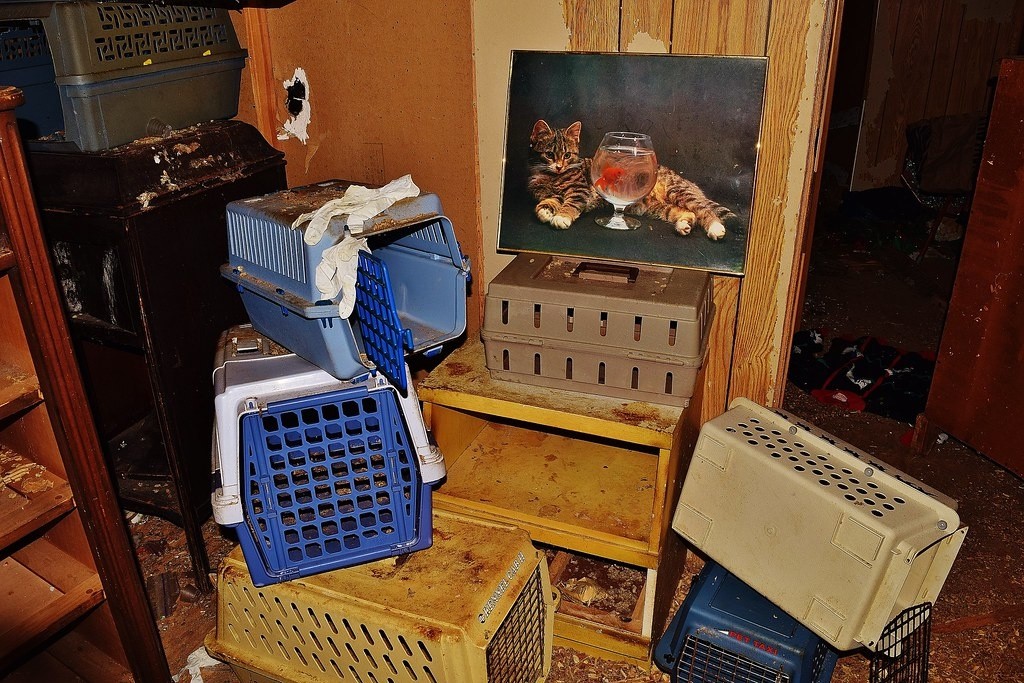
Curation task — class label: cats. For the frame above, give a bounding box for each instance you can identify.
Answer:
[521,119,737,242]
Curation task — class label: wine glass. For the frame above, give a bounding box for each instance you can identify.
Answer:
[590,131,658,230]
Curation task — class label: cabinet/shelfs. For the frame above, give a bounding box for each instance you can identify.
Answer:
[821,1,1024,192]
[1,83,174,683]
[20,117,288,594]
[418,329,710,677]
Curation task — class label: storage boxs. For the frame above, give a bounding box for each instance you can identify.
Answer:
[0,0,251,154]
[203,508,555,683]
[654,397,969,658]
[652,559,838,683]
[209,179,472,587]
[480,253,716,407]
